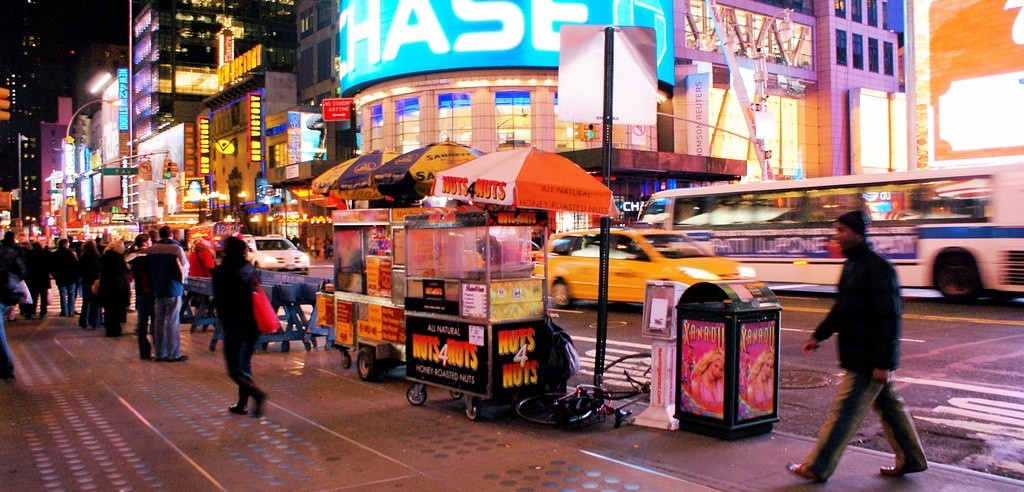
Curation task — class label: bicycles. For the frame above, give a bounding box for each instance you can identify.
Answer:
[513,369,652,433]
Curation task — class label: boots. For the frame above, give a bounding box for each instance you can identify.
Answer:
[239,377,267,417]
[229,394,249,415]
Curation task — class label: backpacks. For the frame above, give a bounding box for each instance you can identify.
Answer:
[535,316,580,380]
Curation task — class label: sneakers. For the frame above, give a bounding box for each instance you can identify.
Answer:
[880,463,928,477]
[786,462,825,483]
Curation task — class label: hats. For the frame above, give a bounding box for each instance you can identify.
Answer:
[838,211,870,236]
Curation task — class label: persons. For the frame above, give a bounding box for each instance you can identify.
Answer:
[188,239,215,315]
[476,235,528,268]
[324,235,333,249]
[690,353,725,408]
[350,229,377,273]
[749,349,774,410]
[0,241,27,379]
[286,234,306,248]
[127,226,191,362]
[532,229,544,251]
[210,236,266,418]
[50,229,133,337]
[0,231,52,323]
[786,210,928,484]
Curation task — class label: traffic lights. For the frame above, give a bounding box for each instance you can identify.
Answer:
[0,87,12,121]
[161,159,173,179]
[172,162,179,178]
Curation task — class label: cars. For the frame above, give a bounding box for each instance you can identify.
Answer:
[531,222,756,309]
[242,232,308,273]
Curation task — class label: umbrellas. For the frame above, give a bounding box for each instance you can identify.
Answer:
[312,138,624,221]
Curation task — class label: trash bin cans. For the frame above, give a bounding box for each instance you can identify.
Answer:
[673,279,784,439]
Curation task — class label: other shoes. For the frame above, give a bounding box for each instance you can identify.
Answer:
[156,355,168,362]
[168,354,187,362]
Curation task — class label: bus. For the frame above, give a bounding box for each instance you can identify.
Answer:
[641,162,1024,307]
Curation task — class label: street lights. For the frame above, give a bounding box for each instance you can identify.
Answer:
[590,25,623,388]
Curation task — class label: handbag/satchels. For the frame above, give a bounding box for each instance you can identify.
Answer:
[18,280,35,305]
[252,271,280,335]
[1,268,27,306]
